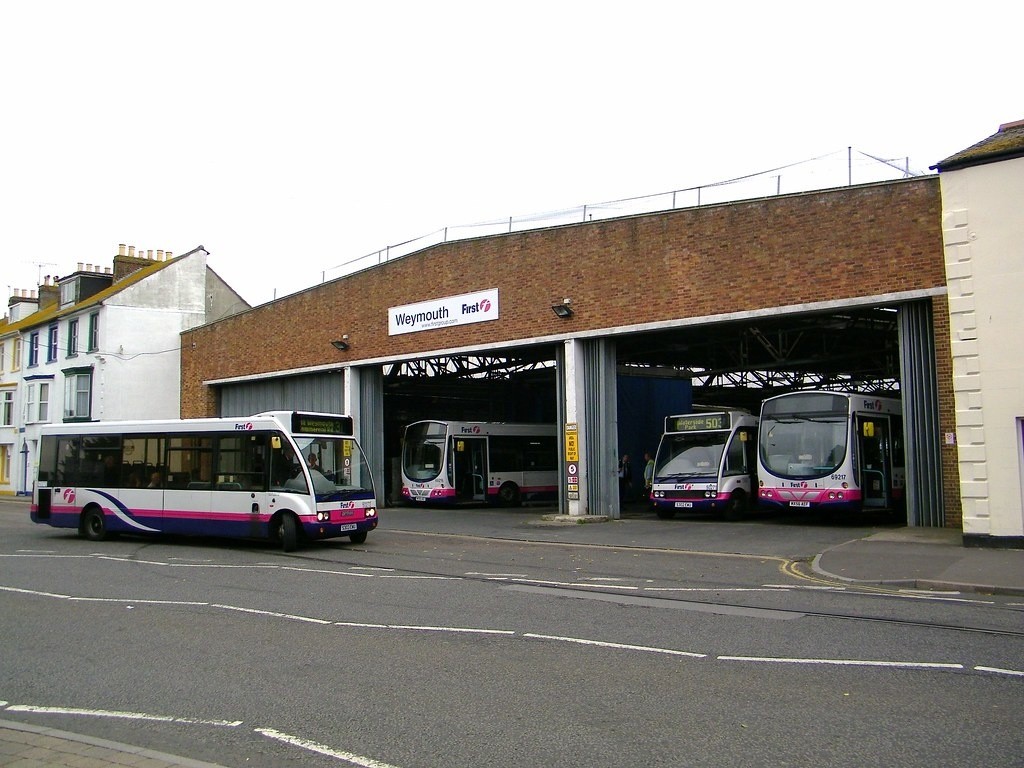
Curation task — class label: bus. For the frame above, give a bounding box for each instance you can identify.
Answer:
[30,410,378,554]
[648,411,798,521]
[402,419,559,509]
[739,389,907,515]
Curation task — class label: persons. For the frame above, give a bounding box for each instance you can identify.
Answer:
[642,452,656,512]
[148,471,164,489]
[277,448,324,490]
[617,454,632,511]
[103,455,121,488]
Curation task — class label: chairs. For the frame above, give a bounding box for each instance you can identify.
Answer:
[123,461,132,473]
[145,463,155,478]
[187,482,242,490]
[132,460,144,473]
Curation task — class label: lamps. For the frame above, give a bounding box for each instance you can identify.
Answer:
[551,299,572,318]
[331,334,349,351]
